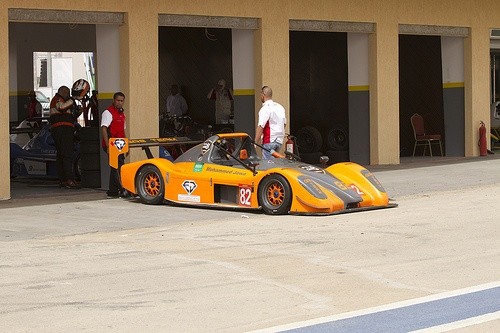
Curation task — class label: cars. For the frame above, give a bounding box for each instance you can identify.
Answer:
[34,90,51,116]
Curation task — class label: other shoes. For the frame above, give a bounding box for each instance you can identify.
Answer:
[59,177,84,189]
[107,193,124,198]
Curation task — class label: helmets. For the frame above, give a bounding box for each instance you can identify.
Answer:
[71,79,90,100]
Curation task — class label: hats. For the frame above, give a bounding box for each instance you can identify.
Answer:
[28,91,37,96]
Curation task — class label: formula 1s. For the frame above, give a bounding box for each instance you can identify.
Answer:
[109,133,399,217]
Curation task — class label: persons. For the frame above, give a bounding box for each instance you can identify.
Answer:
[165,84,189,140]
[48,85,85,189]
[26,90,42,127]
[99,91,128,200]
[254,86,287,162]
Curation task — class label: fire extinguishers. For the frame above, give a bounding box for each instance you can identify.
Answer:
[478,120,488,158]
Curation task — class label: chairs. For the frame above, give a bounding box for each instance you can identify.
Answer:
[212,136,260,166]
[410,113,445,159]
